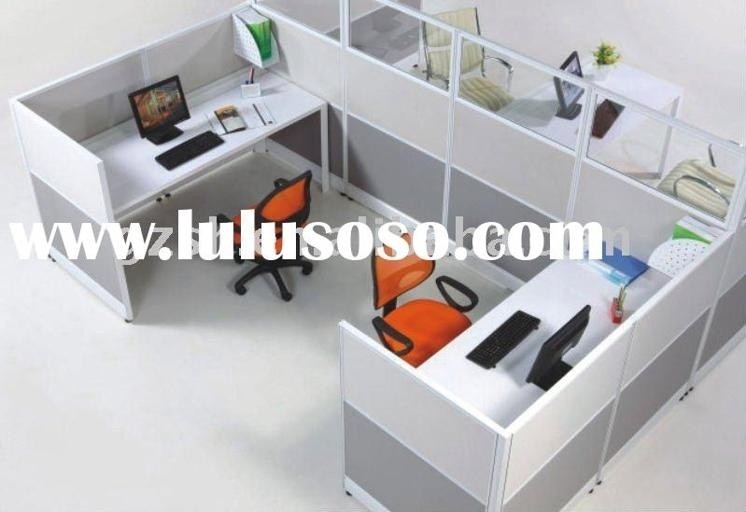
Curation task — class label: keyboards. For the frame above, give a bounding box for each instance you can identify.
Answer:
[155,130,226,172]
[576,98,626,141]
[466,309,541,371]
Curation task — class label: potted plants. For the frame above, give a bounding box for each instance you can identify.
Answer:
[592,42,622,79]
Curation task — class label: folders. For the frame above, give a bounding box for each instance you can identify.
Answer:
[582,241,647,287]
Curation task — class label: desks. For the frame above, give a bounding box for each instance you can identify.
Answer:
[496,61,684,180]
[413,231,733,429]
[78,68,330,322]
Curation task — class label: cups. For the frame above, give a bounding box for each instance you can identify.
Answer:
[609,297,618,313]
[613,308,624,324]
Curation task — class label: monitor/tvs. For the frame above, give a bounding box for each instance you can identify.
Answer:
[525,303,593,391]
[553,50,586,121]
[127,74,192,147]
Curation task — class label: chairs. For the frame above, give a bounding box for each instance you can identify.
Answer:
[422,8,513,112]
[656,140,746,223]
[216,169,313,301]
[371,233,478,369]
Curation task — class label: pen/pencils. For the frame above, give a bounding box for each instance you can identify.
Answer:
[247,66,259,87]
[617,286,628,309]
